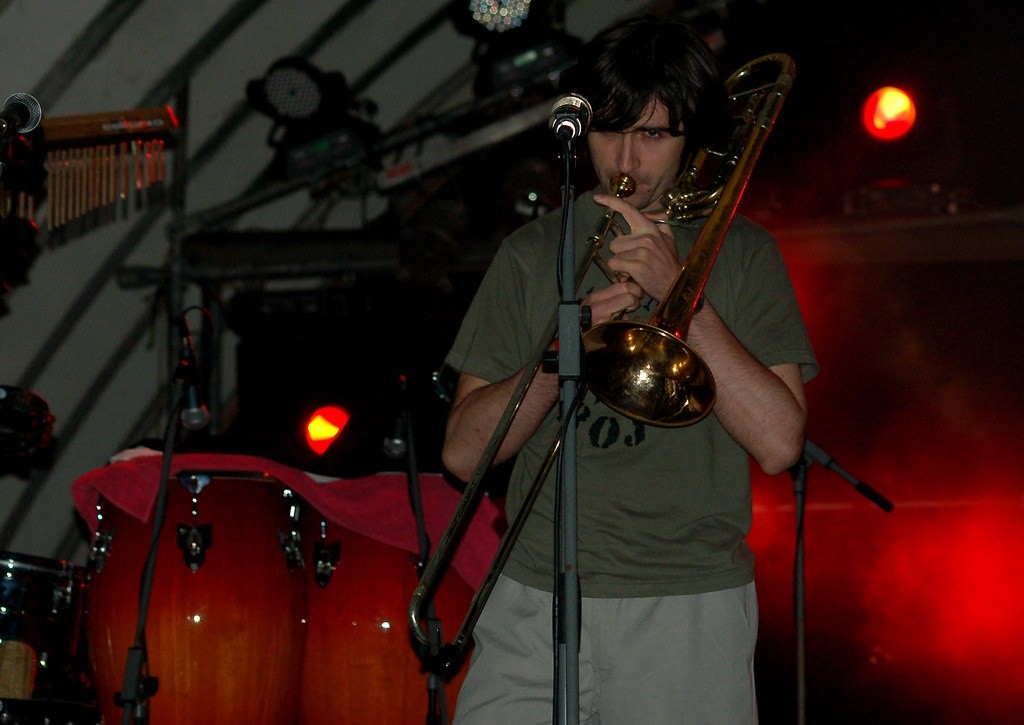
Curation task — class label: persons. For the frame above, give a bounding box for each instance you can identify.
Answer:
[440,11,822,725]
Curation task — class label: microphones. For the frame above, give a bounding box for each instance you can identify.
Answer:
[548,92,593,140]
[0,93,42,133]
[178,317,211,430]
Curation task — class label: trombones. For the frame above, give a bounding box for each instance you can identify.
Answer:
[406,52,802,674]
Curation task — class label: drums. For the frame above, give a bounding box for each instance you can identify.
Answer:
[290,473,499,725]
[0,552,102,725]
[90,466,299,724]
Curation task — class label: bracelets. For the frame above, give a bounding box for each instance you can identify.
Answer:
[693,291,705,315]
